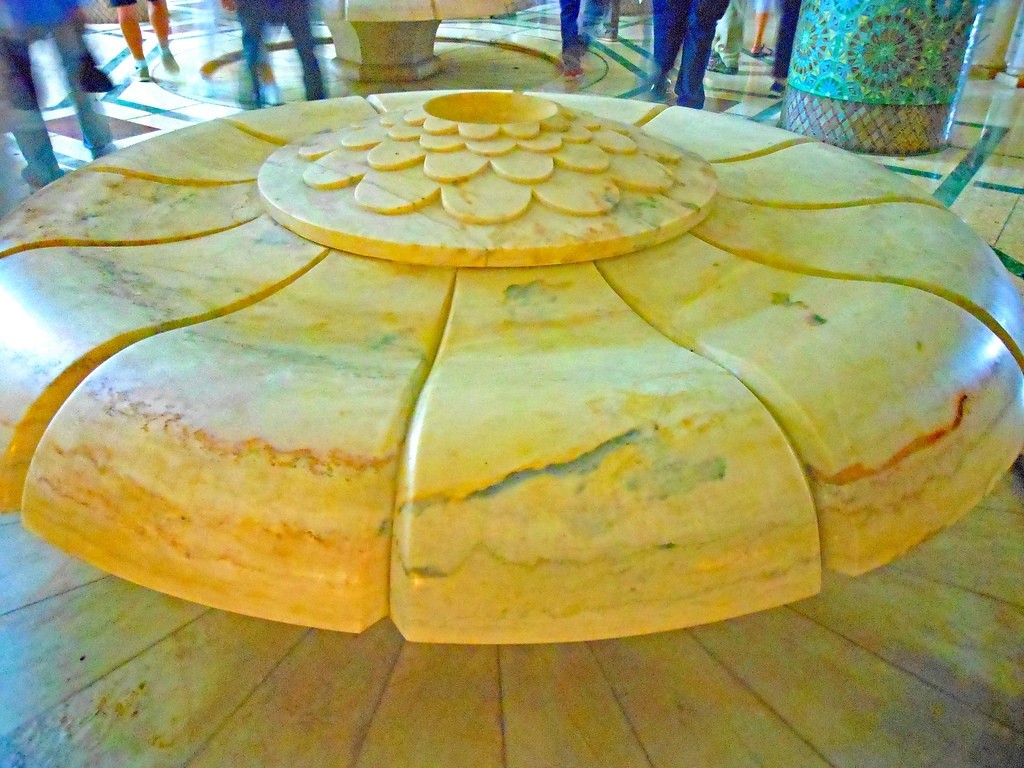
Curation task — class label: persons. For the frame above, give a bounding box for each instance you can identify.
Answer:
[769,0,802,98]
[652,0,731,109]
[0,0,118,190]
[749,1,776,57]
[110,0,181,82]
[705,0,748,75]
[559,0,612,82]
[220,0,328,111]
[593,0,621,41]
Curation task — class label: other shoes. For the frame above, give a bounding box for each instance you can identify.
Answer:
[161,54,179,73]
[79,67,112,92]
[137,66,149,81]
[561,60,583,81]
[751,45,775,56]
[594,27,617,41]
[578,34,588,57]
[260,85,281,105]
[706,55,720,71]
[22,167,64,187]
[767,83,785,97]
[721,67,737,75]
